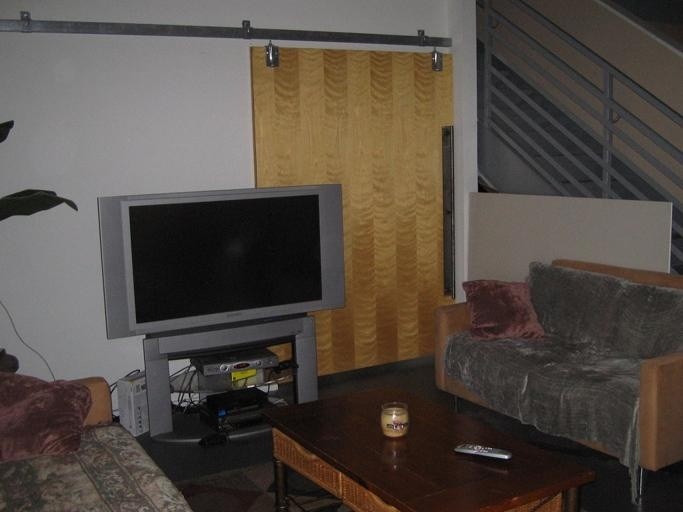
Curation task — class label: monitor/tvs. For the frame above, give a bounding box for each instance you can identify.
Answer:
[96,184,346,341]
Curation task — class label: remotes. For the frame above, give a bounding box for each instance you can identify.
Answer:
[454,442,513,461]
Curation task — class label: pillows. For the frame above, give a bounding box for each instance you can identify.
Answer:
[1,377,95,465]
[461,276,540,340]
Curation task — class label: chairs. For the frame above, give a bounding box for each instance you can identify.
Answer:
[1,374,191,512]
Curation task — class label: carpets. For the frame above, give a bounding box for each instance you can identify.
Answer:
[174,460,350,510]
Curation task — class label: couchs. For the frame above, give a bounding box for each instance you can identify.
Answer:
[432,258,683,498]
